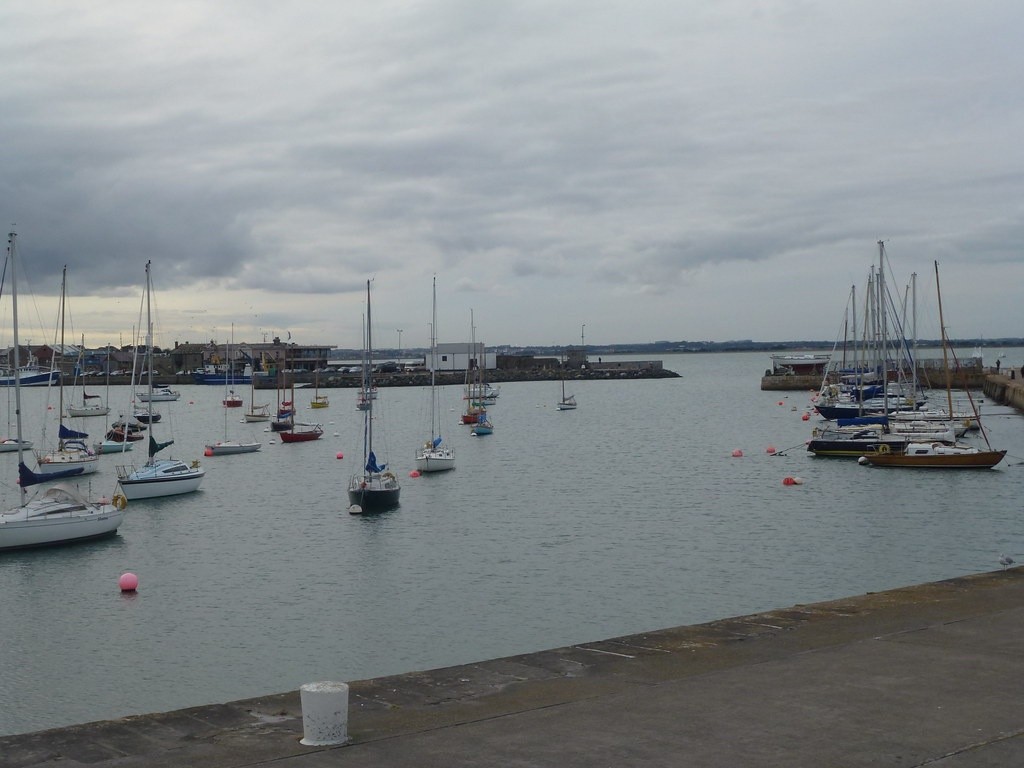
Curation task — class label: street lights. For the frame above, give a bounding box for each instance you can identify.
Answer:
[581,323,586,345]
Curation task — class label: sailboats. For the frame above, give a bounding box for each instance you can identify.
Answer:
[865,257,1008,469]
[345,279,402,517]
[310,344,332,409]
[806,238,984,457]
[115,258,209,501]
[469,340,495,437]
[414,271,457,472]
[0,221,125,554]
[461,307,501,424]
[0,321,325,478]
[356,278,378,400]
[357,313,373,412]
[556,345,578,411]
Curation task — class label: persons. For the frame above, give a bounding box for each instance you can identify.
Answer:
[996,359,1000,375]
[599,357,601,363]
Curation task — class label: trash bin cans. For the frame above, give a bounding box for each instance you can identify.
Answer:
[1011,369,1015,379]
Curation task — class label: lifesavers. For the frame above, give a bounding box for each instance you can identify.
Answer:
[879,444,890,454]
[382,472,395,477]
[830,388,838,396]
[113,493,126,509]
